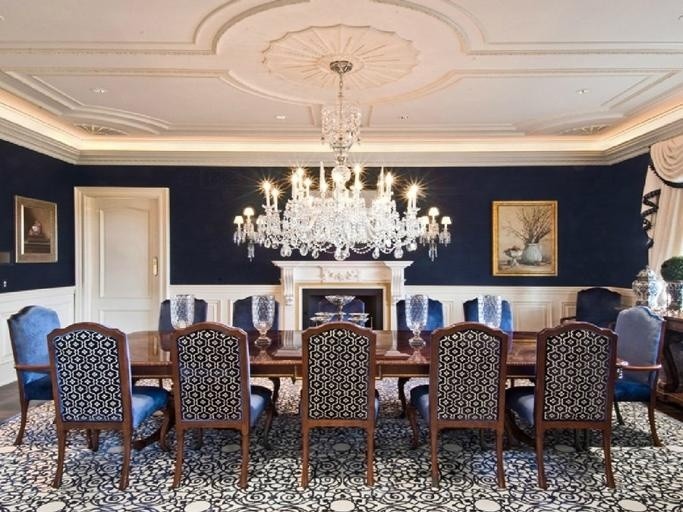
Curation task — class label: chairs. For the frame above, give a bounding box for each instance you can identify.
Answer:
[158,297,208,389]
[46,322,169,491]
[395,293,445,406]
[6,304,61,443]
[584,307,665,450]
[407,321,509,489]
[167,322,274,488]
[233,294,279,414]
[462,296,517,390]
[506,322,618,489]
[316,297,365,328]
[301,322,380,488]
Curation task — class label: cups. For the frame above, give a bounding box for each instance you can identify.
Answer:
[167,291,193,331]
[477,291,501,331]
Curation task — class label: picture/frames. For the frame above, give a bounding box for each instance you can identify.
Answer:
[14,195,59,264]
[492,200,558,277]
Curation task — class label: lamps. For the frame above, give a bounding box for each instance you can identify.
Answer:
[232,62,452,263]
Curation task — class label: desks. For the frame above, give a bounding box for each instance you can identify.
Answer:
[85,329,627,449]
[651,308,683,407]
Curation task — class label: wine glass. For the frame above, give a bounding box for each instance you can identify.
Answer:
[400,294,429,342]
[407,344,426,364]
[249,294,275,344]
[254,345,274,364]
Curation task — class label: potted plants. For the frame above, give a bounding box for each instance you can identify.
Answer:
[660,256,683,308]
